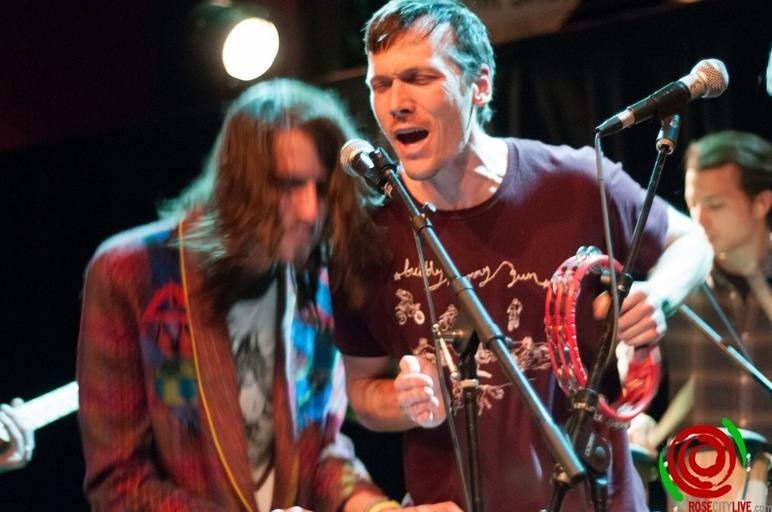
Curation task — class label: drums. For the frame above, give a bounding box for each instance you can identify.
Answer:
[665,425,770,512]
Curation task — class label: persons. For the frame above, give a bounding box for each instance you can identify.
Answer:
[321,1,715,512]
[621,131,771,510]
[78,79,463,512]
[0,396,36,472]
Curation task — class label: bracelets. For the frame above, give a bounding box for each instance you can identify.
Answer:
[367,497,402,511]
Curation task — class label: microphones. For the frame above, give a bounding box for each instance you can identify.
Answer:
[339,139,398,199]
[595,58,729,138]
